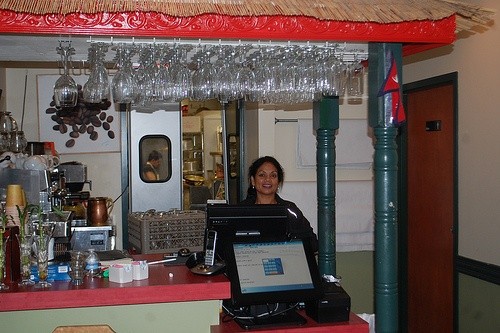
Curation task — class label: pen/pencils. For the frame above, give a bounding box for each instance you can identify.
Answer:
[148,258,176,264]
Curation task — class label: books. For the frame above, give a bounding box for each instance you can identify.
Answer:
[85,249,133,266]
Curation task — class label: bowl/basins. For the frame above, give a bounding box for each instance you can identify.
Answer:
[184,175,205,185]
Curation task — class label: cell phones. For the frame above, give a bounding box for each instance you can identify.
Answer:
[205,231,217,266]
[164,253,178,257]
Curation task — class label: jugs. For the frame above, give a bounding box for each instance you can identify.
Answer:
[64,195,86,218]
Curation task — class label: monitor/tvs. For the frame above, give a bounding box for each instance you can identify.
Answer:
[229,239,323,329]
[205,203,289,264]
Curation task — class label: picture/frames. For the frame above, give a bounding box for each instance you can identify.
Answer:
[36,74,121,155]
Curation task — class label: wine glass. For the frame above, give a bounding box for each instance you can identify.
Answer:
[54,43,365,107]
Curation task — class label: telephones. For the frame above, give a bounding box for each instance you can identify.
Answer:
[191,230,226,274]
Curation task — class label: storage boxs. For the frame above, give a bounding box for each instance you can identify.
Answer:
[127,211,206,254]
[108,261,150,283]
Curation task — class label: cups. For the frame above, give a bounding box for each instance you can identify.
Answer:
[0,111,24,154]
[88,197,114,226]
[5,185,27,226]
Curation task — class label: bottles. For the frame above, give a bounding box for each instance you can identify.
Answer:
[84,249,101,277]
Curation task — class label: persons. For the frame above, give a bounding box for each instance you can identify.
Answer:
[141,150,162,180]
[236,156,318,254]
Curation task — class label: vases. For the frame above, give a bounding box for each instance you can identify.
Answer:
[0,234,51,290]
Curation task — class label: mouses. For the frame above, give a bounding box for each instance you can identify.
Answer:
[178,248,190,256]
